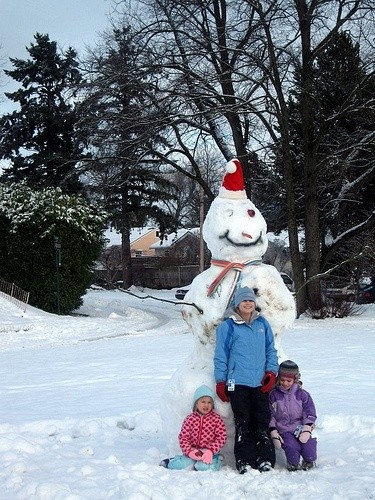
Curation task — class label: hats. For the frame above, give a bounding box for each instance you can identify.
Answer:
[219,160,246,199]
[192,384,215,407]
[235,286,255,308]
[278,360,299,379]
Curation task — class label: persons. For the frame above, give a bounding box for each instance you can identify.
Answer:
[269,360,317,471]
[214,286,279,474]
[159,384,228,470]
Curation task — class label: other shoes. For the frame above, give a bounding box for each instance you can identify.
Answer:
[286,460,315,471]
[258,462,272,472]
[160,458,174,468]
[238,464,248,474]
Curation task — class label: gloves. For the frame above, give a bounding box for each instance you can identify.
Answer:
[216,382,228,402]
[298,425,311,443]
[260,371,275,393]
[270,429,284,450]
[189,448,213,465]
